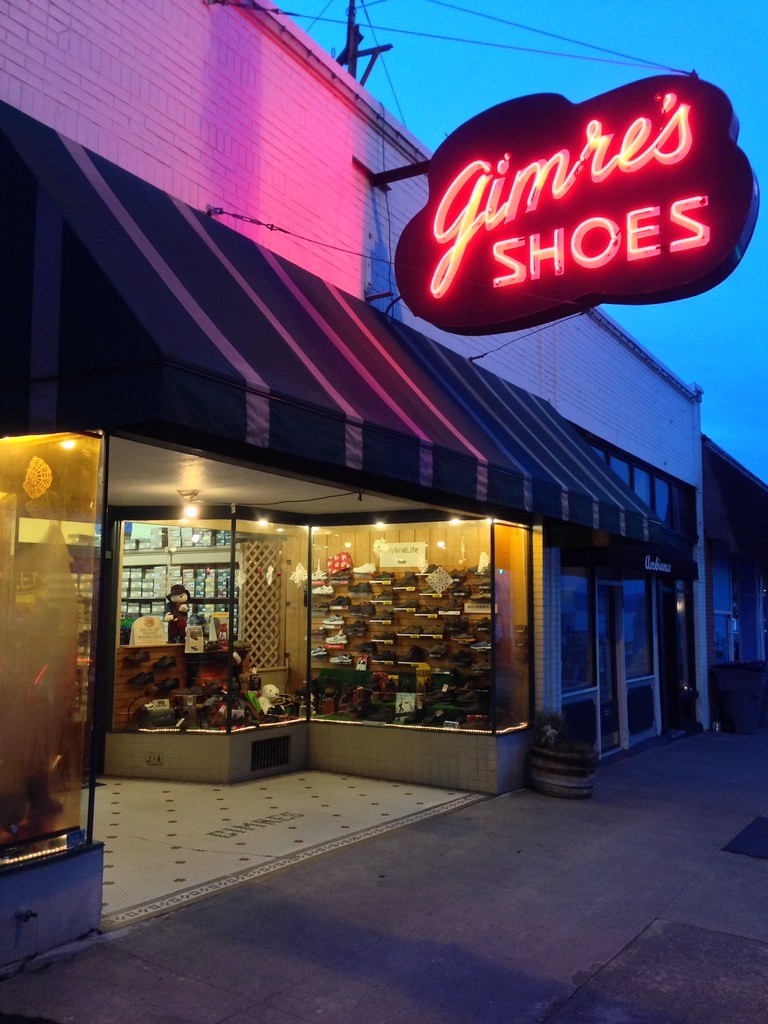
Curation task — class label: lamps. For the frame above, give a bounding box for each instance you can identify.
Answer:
[178,490,200,518]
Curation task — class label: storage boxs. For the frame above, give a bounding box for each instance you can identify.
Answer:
[151,527,167,536]
[124,540,139,549]
[182,538,193,546]
[168,537,181,547]
[70,565,238,642]
[181,527,193,538]
[150,535,167,548]
[168,526,180,537]
[139,539,151,549]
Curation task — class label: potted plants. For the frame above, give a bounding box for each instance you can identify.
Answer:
[524,707,598,800]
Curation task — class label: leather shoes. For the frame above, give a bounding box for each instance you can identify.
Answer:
[154,656,176,669]
[129,672,152,685]
[155,678,178,690]
[124,649,150,663]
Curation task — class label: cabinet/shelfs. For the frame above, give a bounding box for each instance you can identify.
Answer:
[68,543,242,645]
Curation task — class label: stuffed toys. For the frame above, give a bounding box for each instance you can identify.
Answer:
[163,585,191,643]
[258,684,279,716]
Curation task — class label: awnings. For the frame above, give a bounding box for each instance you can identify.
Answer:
[1,97,681,547]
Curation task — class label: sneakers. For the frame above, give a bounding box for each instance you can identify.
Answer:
[309,572,494,726]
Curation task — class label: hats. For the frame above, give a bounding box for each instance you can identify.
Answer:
[166,585,190,601]
